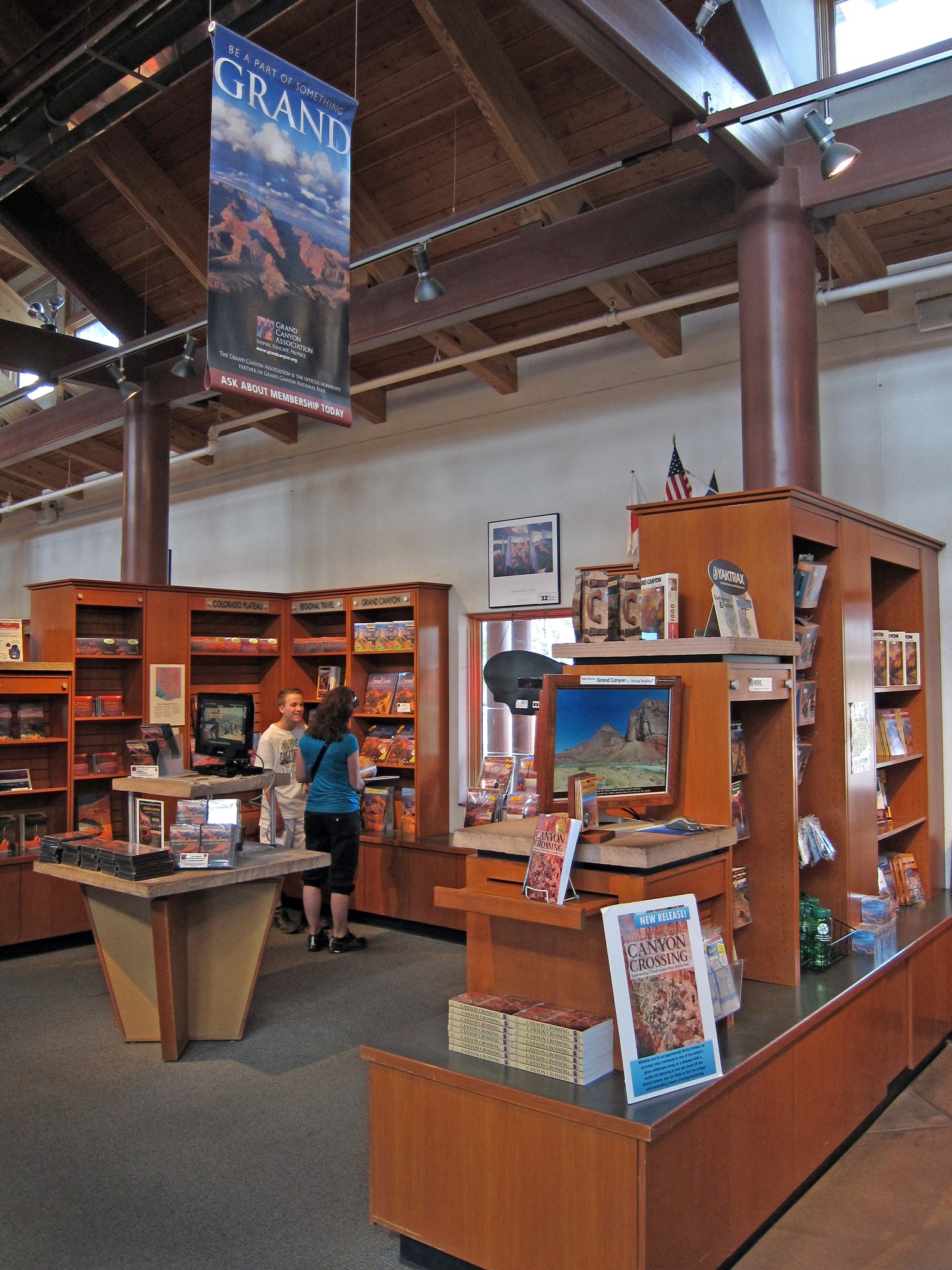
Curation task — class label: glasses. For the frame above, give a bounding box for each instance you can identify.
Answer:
[351,690,357,703]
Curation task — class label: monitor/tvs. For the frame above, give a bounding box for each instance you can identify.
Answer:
[537,674,682,822]
[193,691,255,768]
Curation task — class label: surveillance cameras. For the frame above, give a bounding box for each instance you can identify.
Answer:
[49,501,58,509]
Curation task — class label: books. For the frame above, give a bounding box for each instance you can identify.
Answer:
[38,695,262,881]
[878,851,926,919]
[730,720,753,930]
[522,813,582,905]
[354,621,415,834]
[307,666,340,726]
[875,707,917,756]
[464,754,539,827]
[873,630,920,687]
[877,769,893,826]
[0,702,47,853]
[448,989,614,1085]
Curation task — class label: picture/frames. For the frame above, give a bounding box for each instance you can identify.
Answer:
[487,513,561,608]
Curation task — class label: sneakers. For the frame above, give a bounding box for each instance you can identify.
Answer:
[328,927,368,954]
[307,931,330,952]
[300,911,330,929]
[273,907,299,934]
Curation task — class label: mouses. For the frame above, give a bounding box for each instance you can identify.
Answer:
[666,816,704,831]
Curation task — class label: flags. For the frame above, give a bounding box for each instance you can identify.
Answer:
[627,472,649,569]
[664,444,693,501]
[704,472,719,496]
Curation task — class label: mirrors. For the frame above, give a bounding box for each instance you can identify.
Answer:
[466,608,576,788]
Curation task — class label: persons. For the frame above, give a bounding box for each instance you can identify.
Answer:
[295,687,369,953]
[254,687,330,933]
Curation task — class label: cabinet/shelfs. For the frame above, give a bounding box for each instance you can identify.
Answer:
[0,486,952,1270]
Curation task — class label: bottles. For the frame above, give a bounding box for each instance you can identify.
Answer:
[798,890,833,968]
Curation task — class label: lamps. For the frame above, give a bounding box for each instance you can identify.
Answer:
[800,93,861,183]
[692,0,729,46]
[27,294,64,332]
[171,330,201,379]
[105,357,142,403]
[412,240,444,302]
